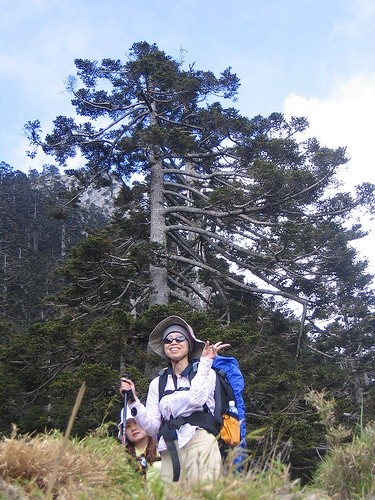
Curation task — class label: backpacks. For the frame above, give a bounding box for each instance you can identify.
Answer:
[159,356,247,472]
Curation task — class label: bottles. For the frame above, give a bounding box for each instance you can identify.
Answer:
[227,401,238,415]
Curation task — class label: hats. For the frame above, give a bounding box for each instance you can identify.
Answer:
[149,316,207,359]
[117,408,135,442]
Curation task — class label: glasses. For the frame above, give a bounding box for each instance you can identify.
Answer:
[163,336,186,343]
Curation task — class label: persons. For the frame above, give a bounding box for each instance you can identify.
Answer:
[120,316,230,500]
[117,406,162,482]
